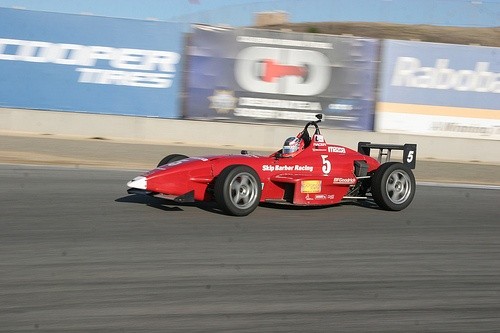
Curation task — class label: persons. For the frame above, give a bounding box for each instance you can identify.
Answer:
[282,136,300,154]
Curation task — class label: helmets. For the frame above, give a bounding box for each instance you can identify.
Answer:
[283,137,300,153]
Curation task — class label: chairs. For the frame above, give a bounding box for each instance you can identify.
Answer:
[299,131,310,149]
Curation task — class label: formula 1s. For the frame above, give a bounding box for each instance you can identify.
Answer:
[126,113,417,216]
